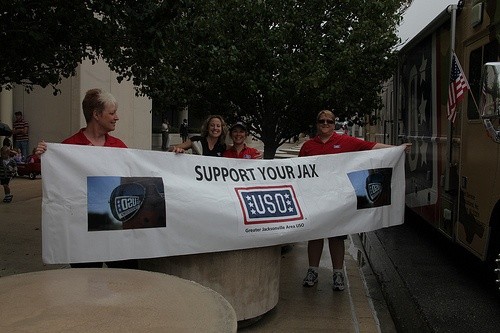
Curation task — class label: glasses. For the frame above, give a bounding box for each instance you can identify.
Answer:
[109,182,165,223]
[318,120,334,124]
[364,172,392,204]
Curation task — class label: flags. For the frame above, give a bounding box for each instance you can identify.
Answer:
[446,52,470,123]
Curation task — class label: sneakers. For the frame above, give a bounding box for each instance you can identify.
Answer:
[333,271,345,291]
[302,268,318,287]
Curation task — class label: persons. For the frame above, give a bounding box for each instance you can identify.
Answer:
[222,121,262,159]
[33,87,131,268]
[162,118,171,151]
[171,115,263,159]
[13,111,29,161]
[298,109,413,291]
[180,119,188,143]
[0,138,17,202]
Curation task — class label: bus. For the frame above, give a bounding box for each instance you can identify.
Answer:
[363,1,499,263]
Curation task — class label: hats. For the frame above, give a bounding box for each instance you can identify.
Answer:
[230,123,247,131]
[15,111,22,116]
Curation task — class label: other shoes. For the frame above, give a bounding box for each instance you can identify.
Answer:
[3,194,14,203]
